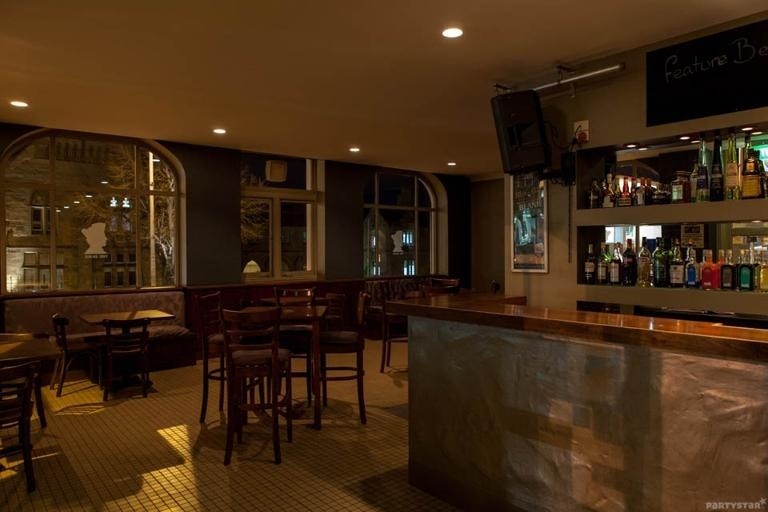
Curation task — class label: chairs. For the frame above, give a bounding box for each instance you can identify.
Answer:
[2,313,153,493]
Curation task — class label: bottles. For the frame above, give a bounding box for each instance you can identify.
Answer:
[521,208,544,244]
[579,126,768,294]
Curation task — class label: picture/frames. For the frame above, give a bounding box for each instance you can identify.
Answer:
[508,173,551,275]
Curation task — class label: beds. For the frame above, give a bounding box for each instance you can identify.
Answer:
[77,307,176,392]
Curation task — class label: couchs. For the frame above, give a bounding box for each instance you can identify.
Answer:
[2,290,194,367]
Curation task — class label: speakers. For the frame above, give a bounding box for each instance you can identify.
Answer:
[490,90,551,174]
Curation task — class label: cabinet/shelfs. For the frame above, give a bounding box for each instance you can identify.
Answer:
[574,196,768,317]
